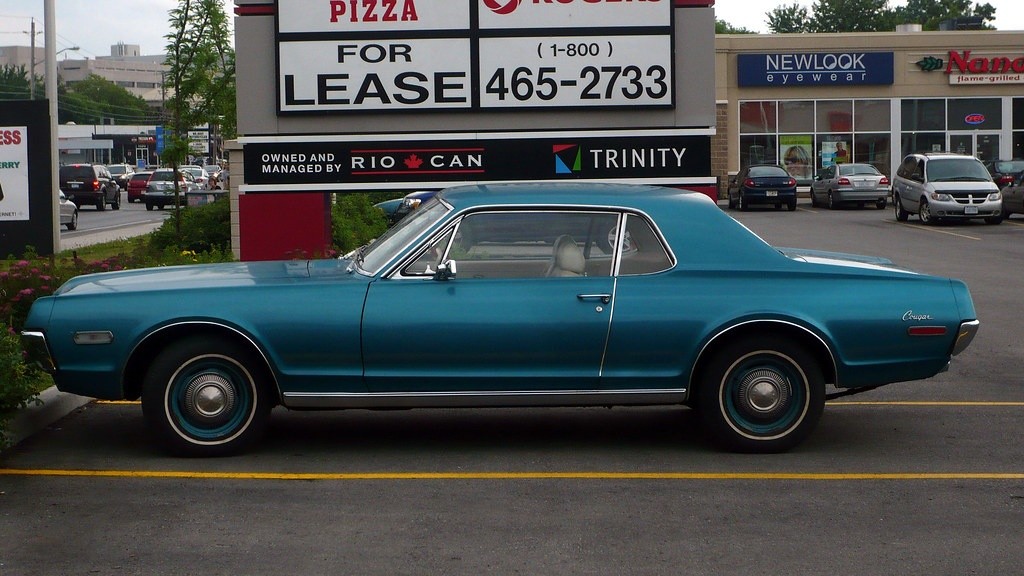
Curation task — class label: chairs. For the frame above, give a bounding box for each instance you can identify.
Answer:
[550,239,585,277]
[545,234,577,277]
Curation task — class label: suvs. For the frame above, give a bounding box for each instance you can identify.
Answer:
[106,163,134,191]
[145,168,186,210]
[59,163,122,211]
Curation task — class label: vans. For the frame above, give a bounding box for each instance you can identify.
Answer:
[892,153,1002,225]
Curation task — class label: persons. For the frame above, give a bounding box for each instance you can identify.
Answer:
[207,176,222,203]
[784,146,812,178]
[830,142,846,164]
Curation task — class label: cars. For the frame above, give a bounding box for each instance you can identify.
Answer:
[180,165,222,190]
[127,171,152,203]
[59,188,78,230]
[982,159,1024,218]
[728,164,797,211]
[809,163,888,210]
[372,190,638,254]
[17,182,979,459]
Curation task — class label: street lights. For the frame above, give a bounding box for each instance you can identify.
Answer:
[31,47,80,103]
